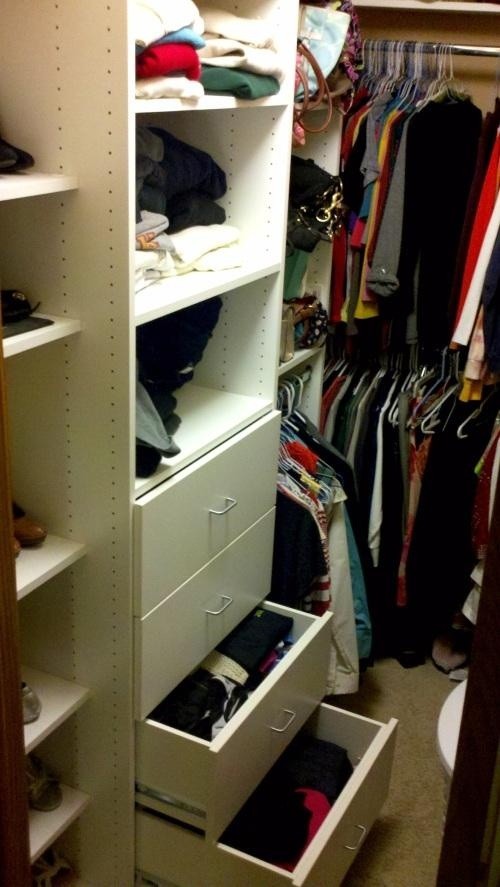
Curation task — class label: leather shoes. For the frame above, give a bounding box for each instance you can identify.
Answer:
[0,136,34,170]
[12,501,47,545]
[0,144,18,168]
[22,681,42,724]
[12,535,22,556]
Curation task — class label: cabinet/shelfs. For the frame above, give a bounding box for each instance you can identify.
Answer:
[0,0,400,887]
[278,98,345,378]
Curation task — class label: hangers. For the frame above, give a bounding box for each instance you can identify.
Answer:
[349,32,470,117]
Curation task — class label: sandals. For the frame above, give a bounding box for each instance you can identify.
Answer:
[25,752,62,809]
[2,288,40,323]
[32,845,74,887]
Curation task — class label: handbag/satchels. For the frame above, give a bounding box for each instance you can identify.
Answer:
[288,301,309,343]
[281,302,296,362]
[286,154,346,259]
[293,0,365,147]
[288,295,328,348]
